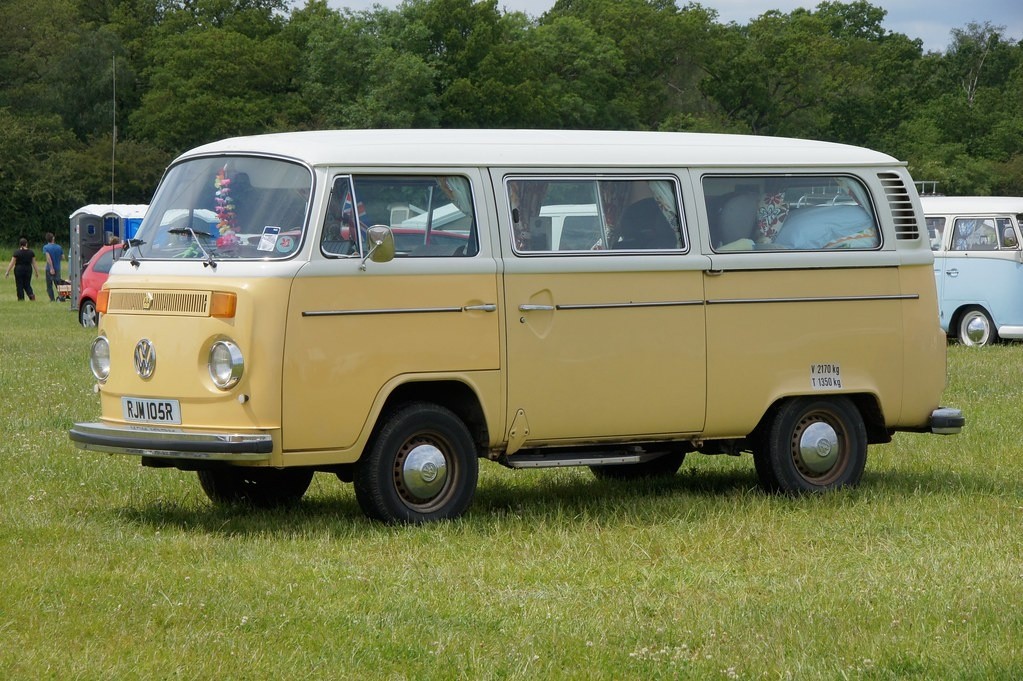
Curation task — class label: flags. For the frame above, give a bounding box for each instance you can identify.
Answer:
[340,189,369,242]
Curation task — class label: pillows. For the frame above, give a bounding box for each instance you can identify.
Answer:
[756,192,790,245]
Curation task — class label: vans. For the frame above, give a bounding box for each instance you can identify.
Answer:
[68,128,966,531]
[776,181,1023,350]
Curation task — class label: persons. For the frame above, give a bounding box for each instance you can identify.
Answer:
[44,233,66,302]
[5,239,39,301]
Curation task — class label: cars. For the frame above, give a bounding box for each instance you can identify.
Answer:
[224,228,513,259]
[77,239,129,329]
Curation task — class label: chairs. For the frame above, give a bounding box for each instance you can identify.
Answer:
[322,240,358,255]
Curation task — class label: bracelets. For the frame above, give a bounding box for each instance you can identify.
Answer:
[6,272,8,274]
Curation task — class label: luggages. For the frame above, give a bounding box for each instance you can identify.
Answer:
[50,275,71,303]
